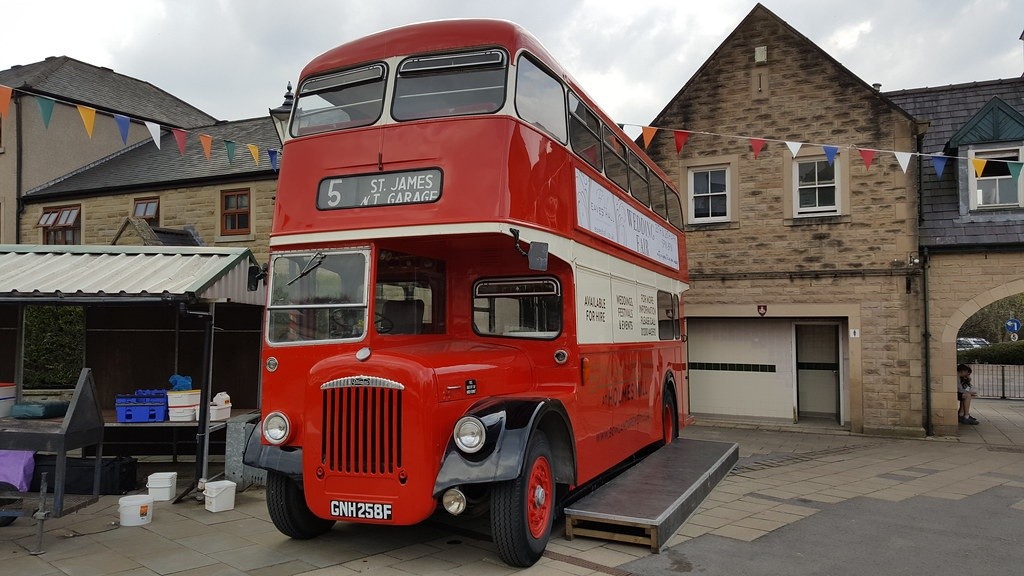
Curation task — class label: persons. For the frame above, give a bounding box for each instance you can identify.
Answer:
[957,364,980,425]
[958,367,977,422]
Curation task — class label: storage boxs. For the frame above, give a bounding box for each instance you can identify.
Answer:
[115,389,237,528]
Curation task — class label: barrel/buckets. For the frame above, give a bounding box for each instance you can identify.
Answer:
[118,493,154,527]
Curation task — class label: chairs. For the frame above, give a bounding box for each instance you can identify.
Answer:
[381,296,426,336]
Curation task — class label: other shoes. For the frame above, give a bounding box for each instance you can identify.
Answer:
[960,415,979,425]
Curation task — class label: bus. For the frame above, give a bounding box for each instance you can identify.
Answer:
[239,19,690,570]
